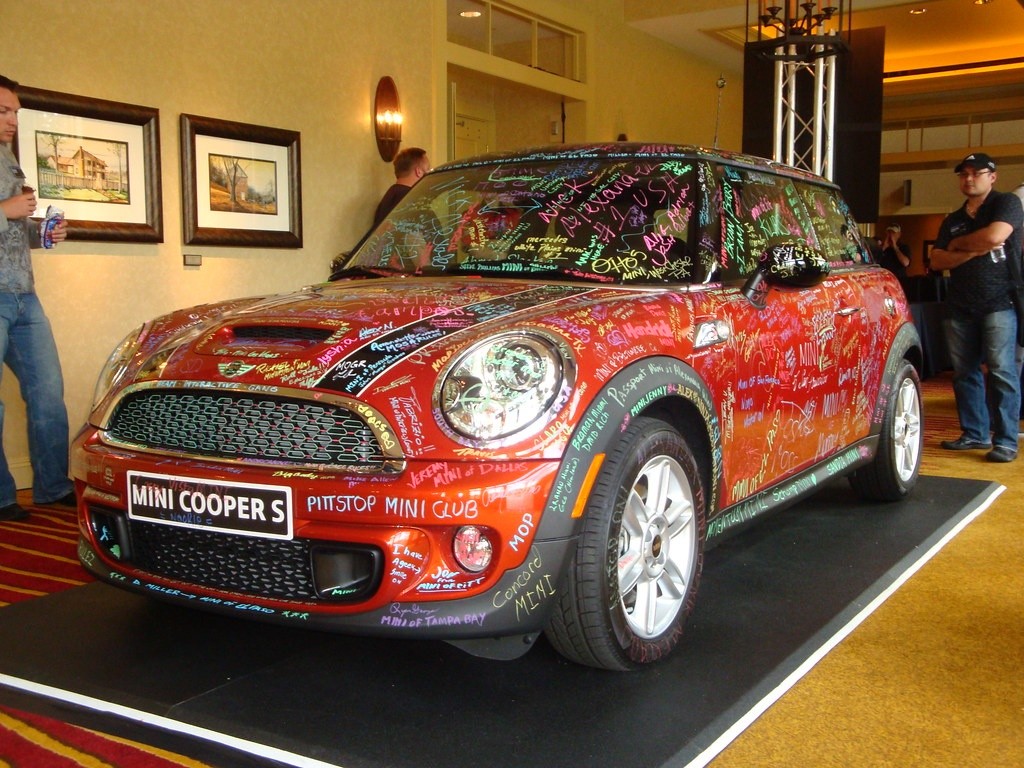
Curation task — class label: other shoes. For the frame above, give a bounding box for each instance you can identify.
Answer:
[33,493,77,507]
[0,503,30,521]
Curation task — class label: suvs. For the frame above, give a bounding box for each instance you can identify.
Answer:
[69,142,925,671]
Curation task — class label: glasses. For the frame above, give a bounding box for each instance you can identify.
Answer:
[957,170,995,177]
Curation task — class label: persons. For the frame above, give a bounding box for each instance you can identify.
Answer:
[877,223,911,299]
[373,147,453,273]
[0,74,82,522]
[929,153,1024,462]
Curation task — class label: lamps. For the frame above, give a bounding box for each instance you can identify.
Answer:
[743,0,852,61]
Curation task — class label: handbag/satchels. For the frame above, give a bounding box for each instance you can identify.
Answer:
[1011,285,1024,347]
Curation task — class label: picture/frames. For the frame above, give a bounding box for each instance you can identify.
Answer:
[9,85,164,244]
[180,113,304,249]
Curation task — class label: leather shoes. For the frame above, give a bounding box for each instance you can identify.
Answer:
[942,438,991,449]
[987,447,1017,461]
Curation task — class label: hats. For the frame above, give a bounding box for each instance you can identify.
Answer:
[954,153,996,173]
[887,223,901,232]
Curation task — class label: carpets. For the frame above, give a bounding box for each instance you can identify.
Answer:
[0,475,1006,768]
